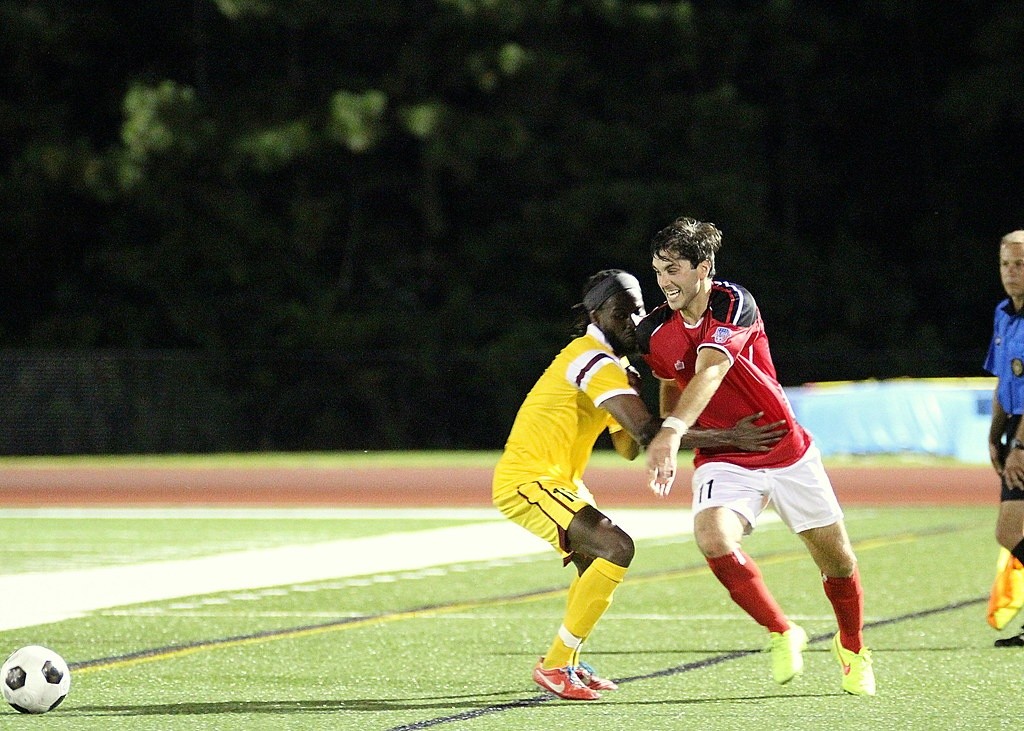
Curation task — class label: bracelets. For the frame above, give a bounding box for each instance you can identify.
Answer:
[661,416,687,436]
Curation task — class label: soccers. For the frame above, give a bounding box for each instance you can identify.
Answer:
[0,645,71,714]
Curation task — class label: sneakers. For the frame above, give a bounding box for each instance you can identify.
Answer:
[533,656,601,700]
[574,662,620,691]
[770,620,808,684]
[994,633,1024,647]
[833,630,877,697]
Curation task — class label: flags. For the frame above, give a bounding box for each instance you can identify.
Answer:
[988,547,1024,633]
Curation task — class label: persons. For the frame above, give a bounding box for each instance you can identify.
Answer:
[491,270,789,700]
[634,217,876,698]
[985,230,1024,647]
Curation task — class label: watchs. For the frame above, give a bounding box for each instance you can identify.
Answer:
[1010,439,1024,450]
